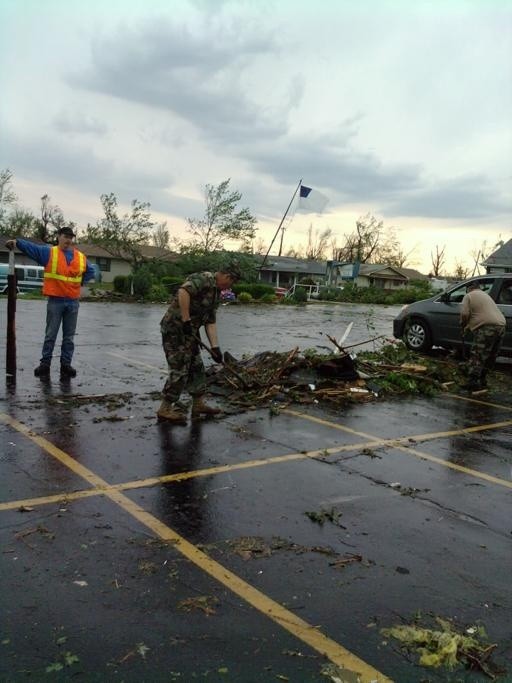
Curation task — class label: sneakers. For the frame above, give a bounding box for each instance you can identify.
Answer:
[459,384,488,395]
[34,365,51,377]
[60,364,77,378]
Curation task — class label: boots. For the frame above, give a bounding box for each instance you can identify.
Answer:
[157,397,187,423]
[191,394,222,418]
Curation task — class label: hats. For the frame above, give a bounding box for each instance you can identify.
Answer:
[58,227,76,237]
[223,261,246,281]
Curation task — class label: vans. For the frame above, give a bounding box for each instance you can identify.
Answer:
[0,262,45,294]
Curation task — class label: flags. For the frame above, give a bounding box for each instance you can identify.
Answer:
[296,178,312,198]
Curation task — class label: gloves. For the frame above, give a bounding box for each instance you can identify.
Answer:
[182,319,194,338]
[211,347,223,364]
[79,286,89,297]
[5,239,16,250]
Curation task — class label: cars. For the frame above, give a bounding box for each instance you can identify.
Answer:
[393,272,512,362]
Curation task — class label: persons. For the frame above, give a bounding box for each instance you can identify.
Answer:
[6,226,94,378]
[456,281,506,394]
[157,263,250,423]
